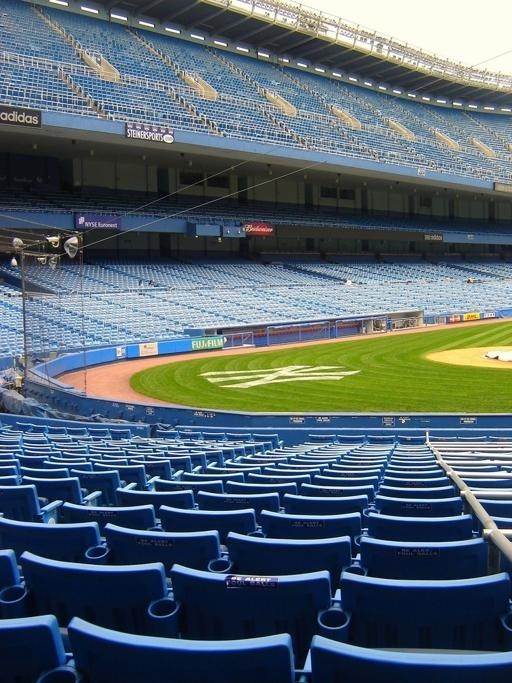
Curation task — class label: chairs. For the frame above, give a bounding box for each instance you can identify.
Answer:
[0,0,512,369]
[0,388,512,682]
